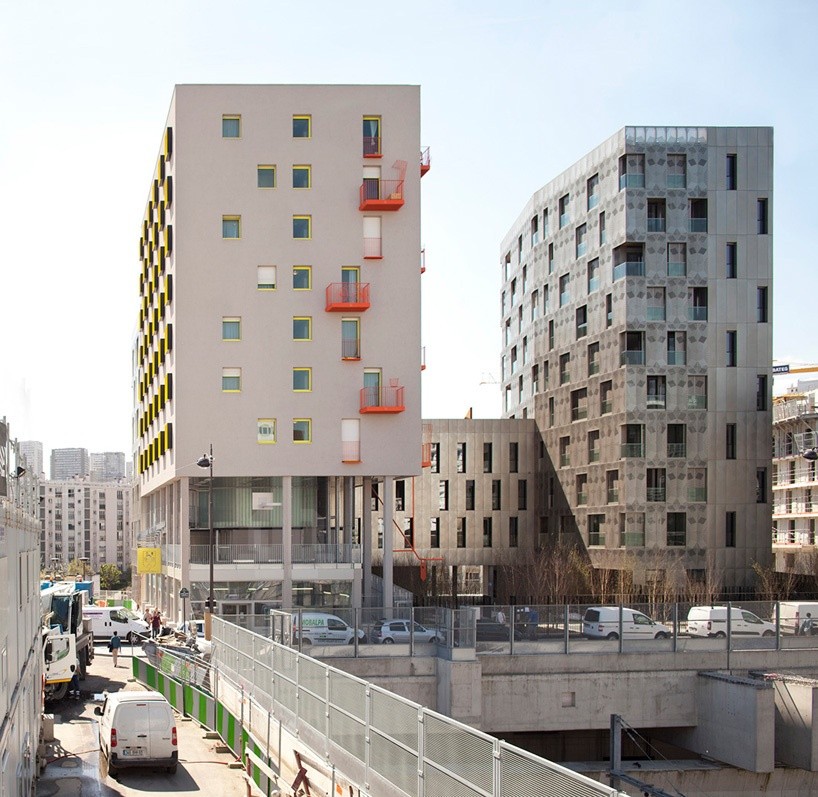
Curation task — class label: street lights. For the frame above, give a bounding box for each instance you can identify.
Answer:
[195,443,215,614]
[78,556,91,582]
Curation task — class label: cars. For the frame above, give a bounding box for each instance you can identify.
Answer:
[453,616,523,646]
[176,620,204,638]
[370,618,446,645]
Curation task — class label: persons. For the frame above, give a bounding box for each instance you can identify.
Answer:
[143,609,152,625]
[150,612,160,639]
[154,608,160,634]
[68,658,80,701]
[110,631,121,667]
[802,613,813,635]
[497,608,506,625]
[528,608,537,622]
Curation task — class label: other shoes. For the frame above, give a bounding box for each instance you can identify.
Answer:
[70,695,74,699]
[75,696,80,700]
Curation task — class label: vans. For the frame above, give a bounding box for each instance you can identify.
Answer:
[82,605,152,646]
[685,605,776,639]
[93,690,178,779]
[292,611,368,646]
[581,606,672,641]
[771,600,818,636]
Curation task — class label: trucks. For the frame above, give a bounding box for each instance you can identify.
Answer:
[38,581,95,714]
